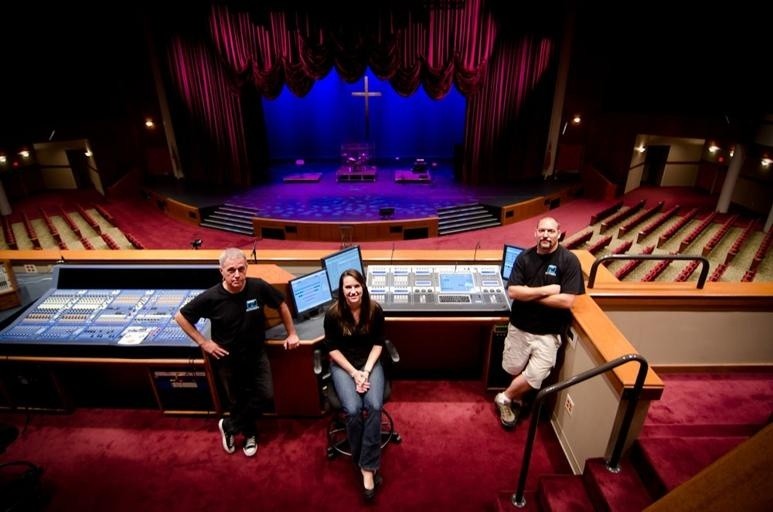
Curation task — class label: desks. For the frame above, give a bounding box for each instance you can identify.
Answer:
[0,347,226,418]
[383,312,513,392]
[266,304,334,420]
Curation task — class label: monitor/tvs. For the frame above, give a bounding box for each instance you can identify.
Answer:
[499,242,526,282]
[320,243,367,294]
[288,267,335,321]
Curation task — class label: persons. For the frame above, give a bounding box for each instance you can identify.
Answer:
[322,269,386,500]
[174,247,300,457]
[494,216,585,427]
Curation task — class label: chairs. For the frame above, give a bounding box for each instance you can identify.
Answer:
[558,198,772,284]
[0,203,146,250]
[313,324,400,487]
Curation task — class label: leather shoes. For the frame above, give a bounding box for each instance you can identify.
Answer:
[372,472,383,486]
[358,467,374,500]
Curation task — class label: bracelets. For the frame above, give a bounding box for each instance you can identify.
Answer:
[361,368,371,373]
[351,369,358,377]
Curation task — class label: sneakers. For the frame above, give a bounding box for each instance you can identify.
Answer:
[510,398,522,415]
[218,418,235,454]
[493,392,517,427]
[243,429,257,457]
